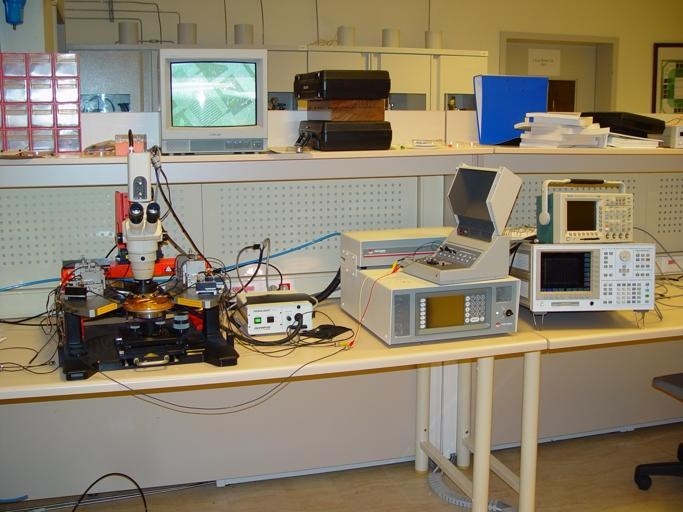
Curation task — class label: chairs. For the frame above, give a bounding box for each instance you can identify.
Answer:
[634,374,682,489]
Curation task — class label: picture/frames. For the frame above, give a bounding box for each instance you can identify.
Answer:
[653,43,683,114]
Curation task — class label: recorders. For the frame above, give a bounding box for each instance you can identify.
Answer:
[536,179,634,244]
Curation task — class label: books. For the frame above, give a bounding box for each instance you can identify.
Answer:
[512,111,666,150]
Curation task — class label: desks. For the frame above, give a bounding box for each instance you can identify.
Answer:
[457,277,683,512]
[1,297,548,512]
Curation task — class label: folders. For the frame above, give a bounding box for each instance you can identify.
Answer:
[473,74,549,145]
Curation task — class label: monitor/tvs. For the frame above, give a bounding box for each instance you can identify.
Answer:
[159,48,268,156]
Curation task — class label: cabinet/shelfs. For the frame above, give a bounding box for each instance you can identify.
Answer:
[267,44,489,145]
